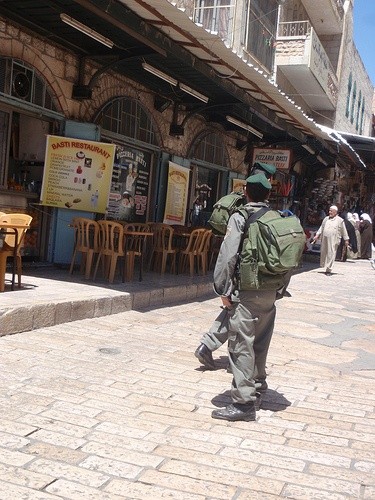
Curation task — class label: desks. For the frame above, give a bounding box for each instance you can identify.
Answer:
[161,230,217,273]
[71,226,154,281]
[0,223,30,292]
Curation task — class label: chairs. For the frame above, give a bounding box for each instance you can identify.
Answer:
[0,212,33,293]
[69,216,214,284]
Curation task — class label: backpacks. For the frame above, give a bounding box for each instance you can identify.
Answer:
[231,206,307,291]
[207,190,246,235]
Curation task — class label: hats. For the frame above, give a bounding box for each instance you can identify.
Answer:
[245,174,273,191]
[253,161,277,175]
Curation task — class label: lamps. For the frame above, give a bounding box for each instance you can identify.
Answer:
[154,96,172,113]
[60,13,113,49]
[317,156,328,166]
[179,83,208,103]
[225,115,263,139]
[142,63,178,87]
[303,145,316,155]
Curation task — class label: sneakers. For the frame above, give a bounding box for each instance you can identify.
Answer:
[211,402,256,421]
[194,343,216,370]
[232,377,268,393]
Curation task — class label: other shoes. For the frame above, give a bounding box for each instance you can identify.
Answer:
[326,272,331,276]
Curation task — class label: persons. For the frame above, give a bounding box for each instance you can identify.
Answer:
[313,205,350,276]
[290,204,304,222]
[338,206,375,262]
[194,161,277,421]
